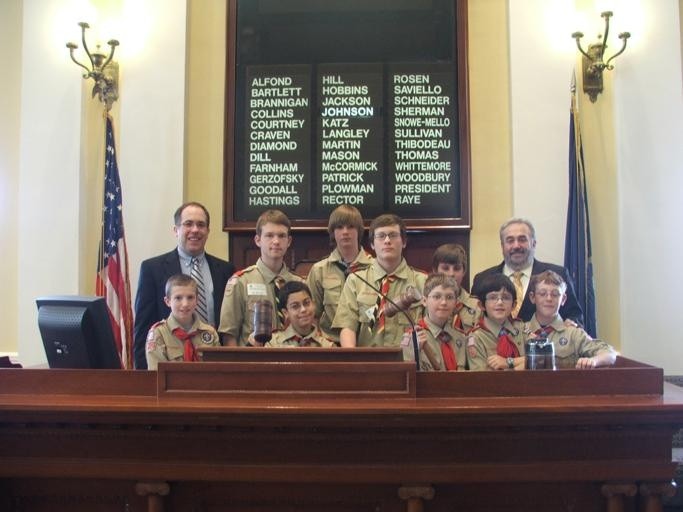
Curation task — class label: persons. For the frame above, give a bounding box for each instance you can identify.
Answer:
[522,269,616,371]
[217,210,306,348]
[464,272,525,372]
[306,204,374,347]
[248,281,341,348]
[470,219,585,330]
[132,203,236,371]
[144,275,221,372]
[331,214,428,348]
[423,243,485,336]
[398,271,468,373]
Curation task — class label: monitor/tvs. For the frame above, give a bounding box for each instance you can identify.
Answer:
[35,295,122,370]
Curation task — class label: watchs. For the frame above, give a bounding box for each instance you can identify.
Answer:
[507,357,514,368]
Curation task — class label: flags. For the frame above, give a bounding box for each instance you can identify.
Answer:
[96,112,133,369]
[563,93,595,341]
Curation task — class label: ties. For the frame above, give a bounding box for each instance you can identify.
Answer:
[191,258,208,325]
[510,273,525,318]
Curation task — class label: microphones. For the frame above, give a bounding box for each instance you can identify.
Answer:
[335,257,421,370]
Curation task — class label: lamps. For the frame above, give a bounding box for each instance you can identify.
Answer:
[565,3,642,103]
[51,1,127,111]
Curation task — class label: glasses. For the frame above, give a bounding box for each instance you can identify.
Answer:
[374,231,400,241]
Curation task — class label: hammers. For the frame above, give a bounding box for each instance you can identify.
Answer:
[383,286,440,371]
[245,300,273,346]
